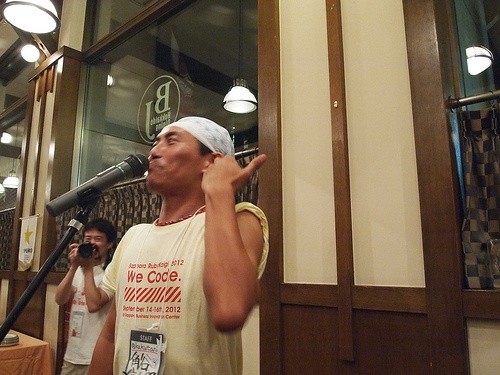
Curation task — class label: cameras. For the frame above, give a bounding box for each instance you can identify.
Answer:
[77,241,100,259]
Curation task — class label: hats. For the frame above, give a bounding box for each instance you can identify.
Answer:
[168,116,234,158]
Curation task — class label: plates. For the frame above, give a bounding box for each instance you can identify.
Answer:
[0,334,19,346]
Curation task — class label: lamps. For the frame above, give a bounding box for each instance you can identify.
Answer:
[3,123,19,188]
[223,0,258,115]
[0,0,60,34]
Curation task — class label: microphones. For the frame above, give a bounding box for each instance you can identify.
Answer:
[45,153,150,217]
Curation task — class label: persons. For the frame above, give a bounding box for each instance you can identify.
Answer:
[86,116,268,375]
[55,217,117,375]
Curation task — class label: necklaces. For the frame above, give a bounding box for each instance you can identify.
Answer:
[153,209,202,227]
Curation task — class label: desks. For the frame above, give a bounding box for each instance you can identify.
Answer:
[0,329,54,375]
